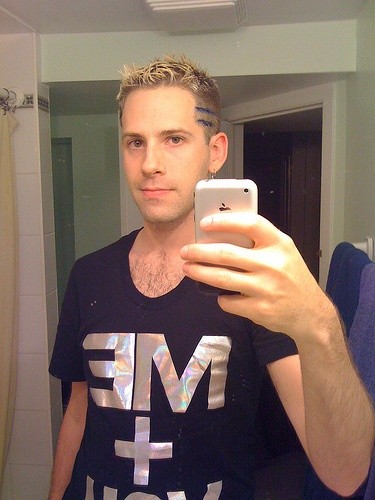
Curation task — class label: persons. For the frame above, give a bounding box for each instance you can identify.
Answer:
[45,52,375,500]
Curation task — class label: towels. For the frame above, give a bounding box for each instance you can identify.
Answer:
[325,241,375,338]
[348,262,375,412]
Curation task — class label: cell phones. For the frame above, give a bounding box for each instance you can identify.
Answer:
[193,178,258,295]
